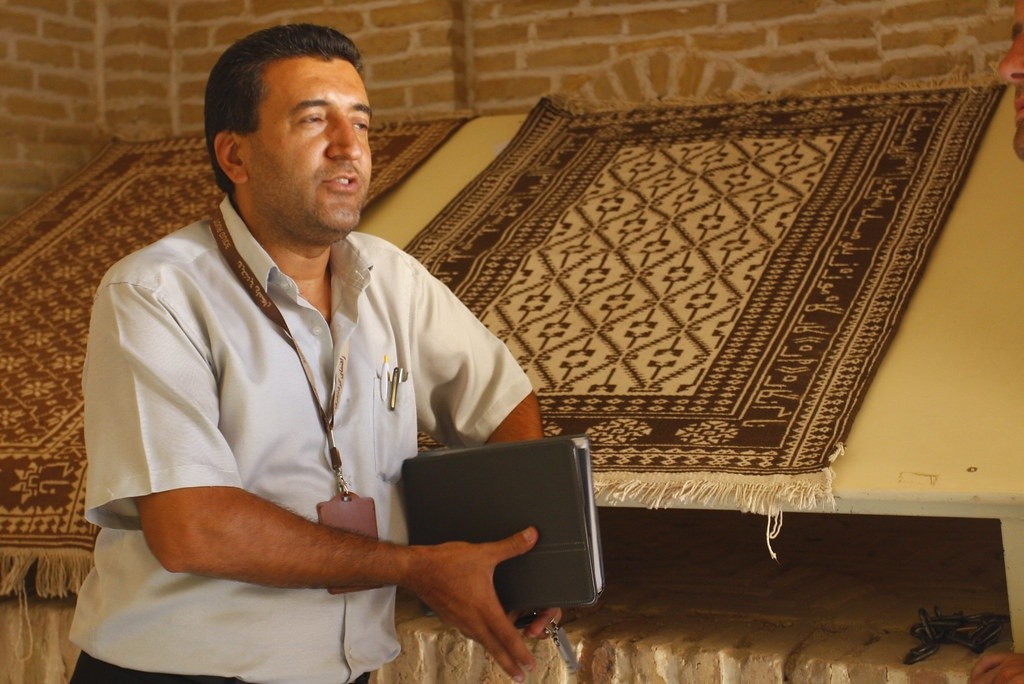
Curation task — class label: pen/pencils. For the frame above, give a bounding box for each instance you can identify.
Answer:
[380,354,405,410]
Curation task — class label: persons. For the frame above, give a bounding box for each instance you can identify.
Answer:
[67,22,565,683]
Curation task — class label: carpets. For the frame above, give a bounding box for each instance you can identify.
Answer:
[0,115,470,600]
[405,84,1004,564]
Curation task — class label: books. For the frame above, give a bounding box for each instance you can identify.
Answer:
[401,433,606,614]
